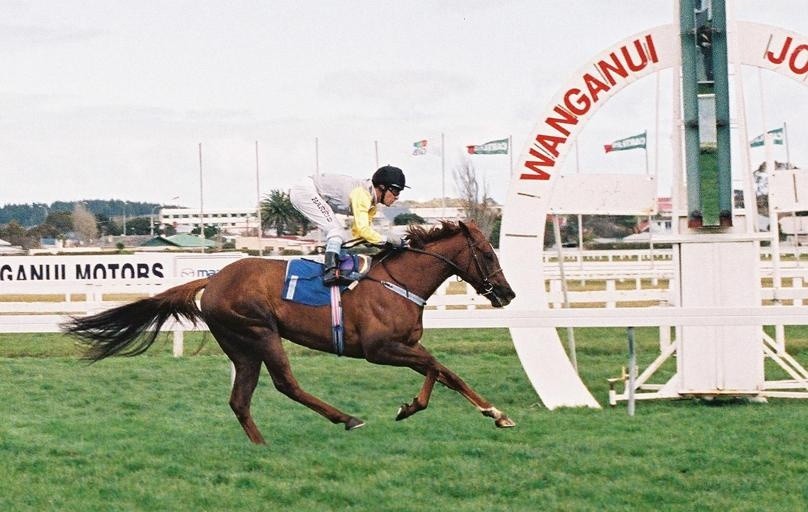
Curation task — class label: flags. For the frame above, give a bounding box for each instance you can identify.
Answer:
[412,140,427,156]
[604,132,647,152]
[466,137,509,153]
[749,126,784,147]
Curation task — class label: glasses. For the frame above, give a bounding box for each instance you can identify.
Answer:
[387,185,401,196]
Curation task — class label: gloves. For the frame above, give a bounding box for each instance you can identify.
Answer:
[386,235,411,248]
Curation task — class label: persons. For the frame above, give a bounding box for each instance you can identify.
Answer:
[289,165,412,286]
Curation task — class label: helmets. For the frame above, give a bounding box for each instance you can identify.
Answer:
[372,165,411,189]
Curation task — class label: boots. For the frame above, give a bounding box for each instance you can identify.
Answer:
[324,251,362,284]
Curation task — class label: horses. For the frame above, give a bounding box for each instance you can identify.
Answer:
[57,218,517,446]
